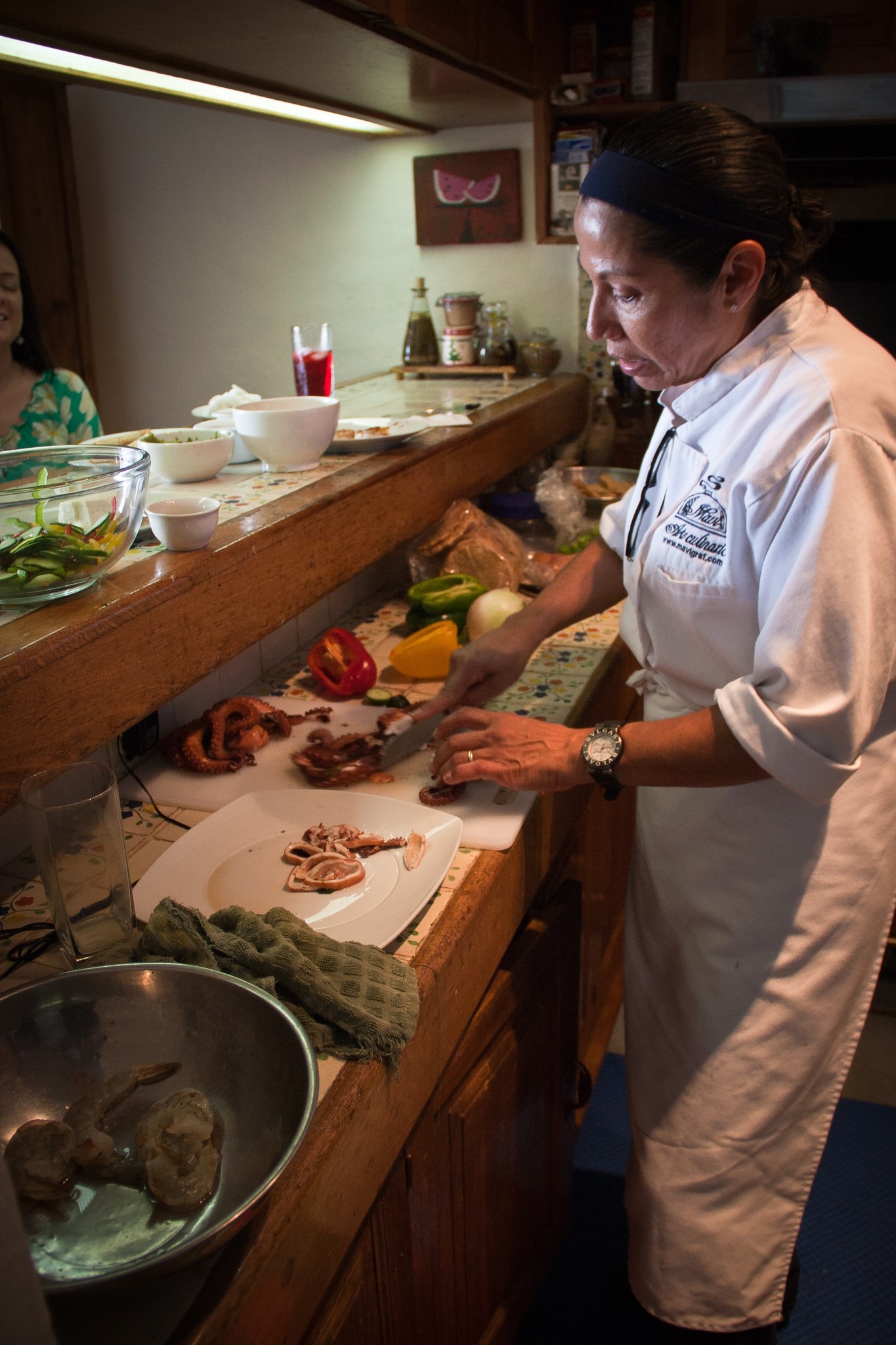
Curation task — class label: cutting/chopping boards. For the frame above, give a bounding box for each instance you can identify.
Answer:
[114,695,550,851]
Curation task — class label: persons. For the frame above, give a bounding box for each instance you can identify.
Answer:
[1,232,106,494]
[408,99,895,1345]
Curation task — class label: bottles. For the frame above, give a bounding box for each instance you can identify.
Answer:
[400,271,565,380]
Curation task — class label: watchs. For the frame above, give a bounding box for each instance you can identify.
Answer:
[581,719,630,802]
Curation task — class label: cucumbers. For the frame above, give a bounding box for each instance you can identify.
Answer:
[0,468,126,592]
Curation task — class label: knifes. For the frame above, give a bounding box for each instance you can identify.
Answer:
[377,707,458,772]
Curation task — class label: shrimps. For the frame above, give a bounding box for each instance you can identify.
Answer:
[5,1062,221,1212]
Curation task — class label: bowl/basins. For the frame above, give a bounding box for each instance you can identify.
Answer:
[558,465,643,517]
[0,393,342,609]
[0,960,318,1297]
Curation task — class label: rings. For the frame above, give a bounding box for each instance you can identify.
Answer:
[467,750,474,762]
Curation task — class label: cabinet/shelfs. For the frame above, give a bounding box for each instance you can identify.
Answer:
[551,788,626,1131]
[297,1146,412,1345]
[529,1,679,245]
[401,871,584,1345]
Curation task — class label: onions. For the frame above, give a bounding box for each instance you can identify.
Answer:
[466,589,521,640]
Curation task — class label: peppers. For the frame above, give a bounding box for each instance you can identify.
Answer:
[404,574,488,633]
[304,628,377,698]
[387,620,459,680]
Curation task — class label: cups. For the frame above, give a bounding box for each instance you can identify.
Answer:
[293,323,336,398]
[17,761,137,970]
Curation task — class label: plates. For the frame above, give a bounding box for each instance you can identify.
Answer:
[134,788,466,958]
[326,416,428,454]
